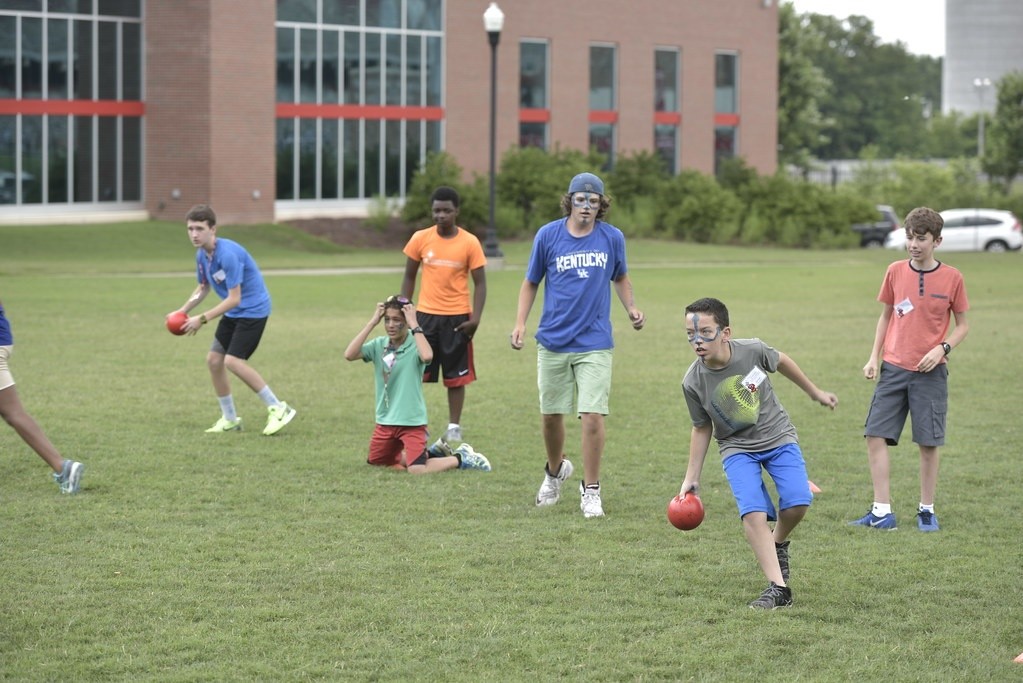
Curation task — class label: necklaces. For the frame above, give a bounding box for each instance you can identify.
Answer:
[382,334,407,407]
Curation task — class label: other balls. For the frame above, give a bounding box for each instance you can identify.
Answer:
[668,492,705,531]
[166,311,188,336]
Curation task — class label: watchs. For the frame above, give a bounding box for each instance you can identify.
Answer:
[411,327,423,335]
[939,341,951,356]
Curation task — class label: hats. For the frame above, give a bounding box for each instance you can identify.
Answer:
[569,173,605,197]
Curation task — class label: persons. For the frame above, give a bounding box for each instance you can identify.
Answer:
[0,303,82,493]
[851,207,970,533]
[511,172,643,518]
[679,297,837,612]
[344,294,491,473]
[401,186,487,441]
[165,205,295,434]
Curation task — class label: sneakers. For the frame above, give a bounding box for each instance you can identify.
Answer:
[205,416,244,433]
[848,509,897,530]
[452,443,491,472]
[53,459,84,493]
[915,508,939,532]
[262,402,296,436]
[446,426,462,442]
[749,580,792,610]
[771,528,790,584]
[430,438,453,457]
[578,479,605,517]
[536,459,573,506]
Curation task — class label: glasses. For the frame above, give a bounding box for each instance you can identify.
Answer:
[387,295,410,304]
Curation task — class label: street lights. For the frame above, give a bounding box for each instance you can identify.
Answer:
[974,78,991,156]
[483,2,504,257]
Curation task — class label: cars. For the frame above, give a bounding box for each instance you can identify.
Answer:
[853,205,900,249]
[884,208,1023,255]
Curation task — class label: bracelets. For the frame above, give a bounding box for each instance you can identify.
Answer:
[199,314,207,324]
